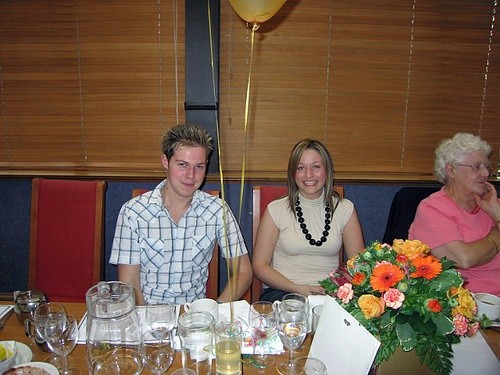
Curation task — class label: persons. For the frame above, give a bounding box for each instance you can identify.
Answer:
[252,139,365,304]
[109,125,253,306]
[408,132,500,299]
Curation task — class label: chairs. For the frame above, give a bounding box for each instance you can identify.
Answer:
[252,185,343,303]
[132,189,219,300]
[28,177,108,301]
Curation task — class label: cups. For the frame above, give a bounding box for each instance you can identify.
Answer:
[289,356,327,375]
[470,291,500,321]
[177,309,214,375]
[311,305,322,342]
[184,298,219,323]
[214,321,243,375]
[272,299,304,322]
[14,288,48,325]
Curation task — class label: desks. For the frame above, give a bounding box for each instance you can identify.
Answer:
[0,299,500,375]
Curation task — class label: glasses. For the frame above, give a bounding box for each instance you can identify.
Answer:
[452,162,494,173]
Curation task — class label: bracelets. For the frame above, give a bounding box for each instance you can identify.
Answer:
[486,236,498,254]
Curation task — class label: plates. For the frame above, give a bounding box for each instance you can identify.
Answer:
[0,362,59,375]
[216,313,227,332]
[15,341,32,365]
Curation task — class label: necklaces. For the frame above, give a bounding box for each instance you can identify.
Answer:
[294,196,332,247]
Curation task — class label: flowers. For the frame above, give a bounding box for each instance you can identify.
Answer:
[319,239,492,375]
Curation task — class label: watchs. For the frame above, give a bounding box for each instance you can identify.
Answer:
[217,300,222,304]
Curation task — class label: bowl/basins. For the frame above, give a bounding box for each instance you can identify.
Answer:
[0,341,18,375]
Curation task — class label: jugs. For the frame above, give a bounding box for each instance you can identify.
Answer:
[85,281,145,375]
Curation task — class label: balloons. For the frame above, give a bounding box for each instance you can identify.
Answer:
[228,0,286,23]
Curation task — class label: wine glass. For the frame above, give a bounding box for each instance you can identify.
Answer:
[171,303,197,375]
[144,298,175,349]
[44,313,80,375]
[139,328,175,375]
[33,302,67,369]
[247,300,279,368]
[280,293,310,357]
[276,309,308,375]
